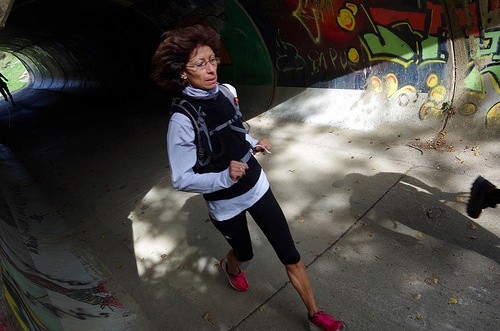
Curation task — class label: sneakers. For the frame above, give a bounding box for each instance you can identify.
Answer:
[467,175,497,219]
[221,258,249,292]
[307,310,346,331]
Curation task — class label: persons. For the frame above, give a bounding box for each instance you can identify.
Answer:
[0,73,15,107]
[465,175,500,219]
[153,24,347,331]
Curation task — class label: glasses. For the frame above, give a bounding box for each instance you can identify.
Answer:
[185,57,223,70]
